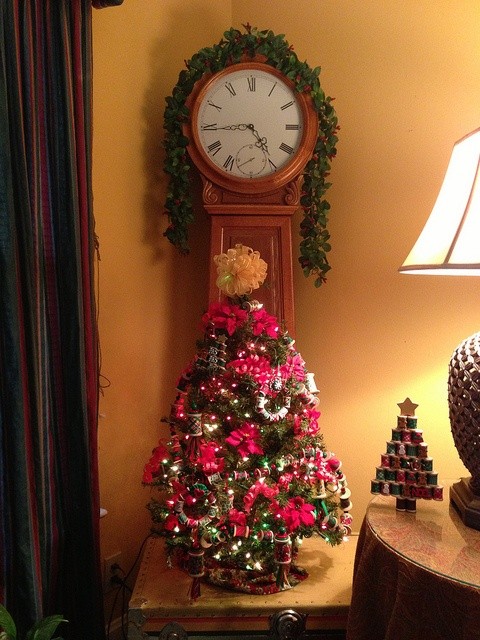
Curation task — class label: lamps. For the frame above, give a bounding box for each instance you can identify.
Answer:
[397,127,480,530]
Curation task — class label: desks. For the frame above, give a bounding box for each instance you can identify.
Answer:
[347,478,480,640]
[129,533,360,639]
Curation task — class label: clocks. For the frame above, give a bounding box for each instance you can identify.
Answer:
[179,42,319,336]
